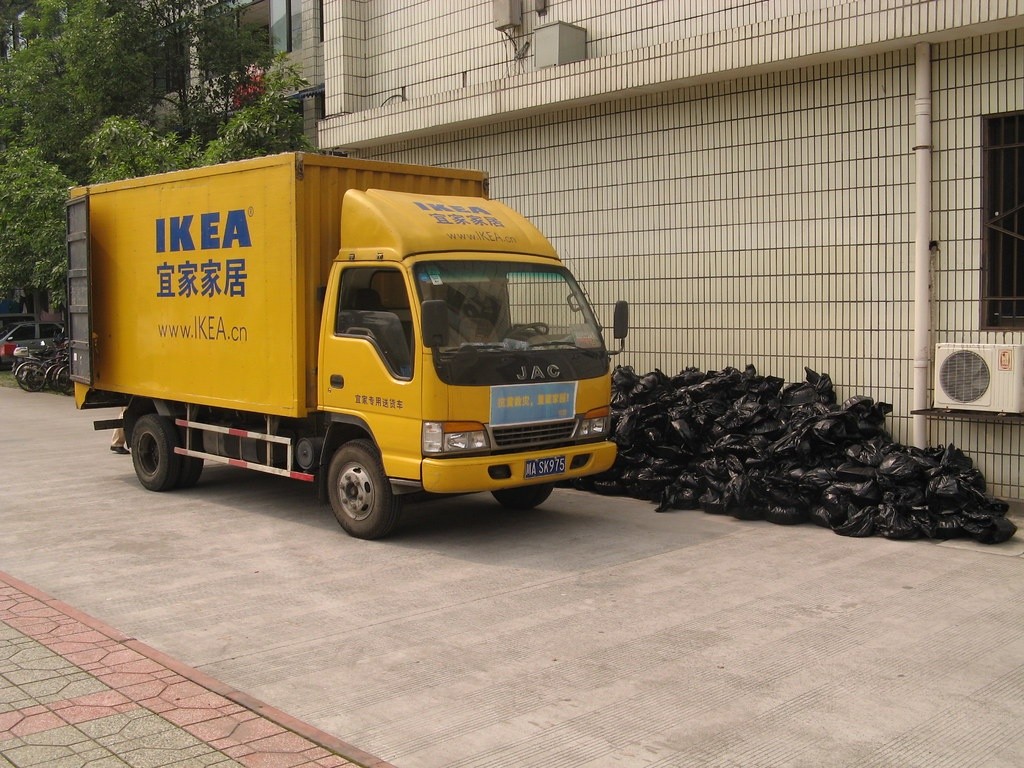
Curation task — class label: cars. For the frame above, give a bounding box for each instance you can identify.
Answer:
[0,321,65,371]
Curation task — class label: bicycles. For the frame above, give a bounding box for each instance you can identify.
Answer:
[1,327,76,397]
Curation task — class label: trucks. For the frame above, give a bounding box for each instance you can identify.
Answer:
[64,150,630,542]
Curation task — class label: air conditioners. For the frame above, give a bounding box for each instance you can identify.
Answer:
[934,342,1024,416]
